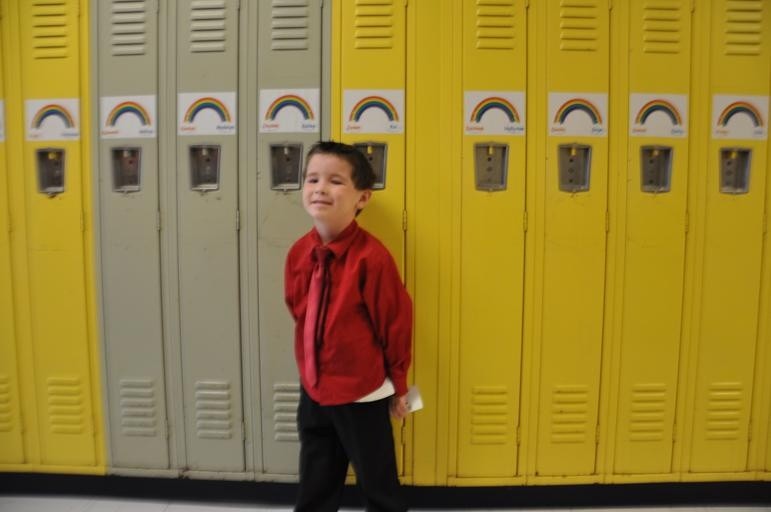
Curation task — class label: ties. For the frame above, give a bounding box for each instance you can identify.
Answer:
[302,244,333,391]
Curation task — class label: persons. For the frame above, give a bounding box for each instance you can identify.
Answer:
[284,142,414,512]
